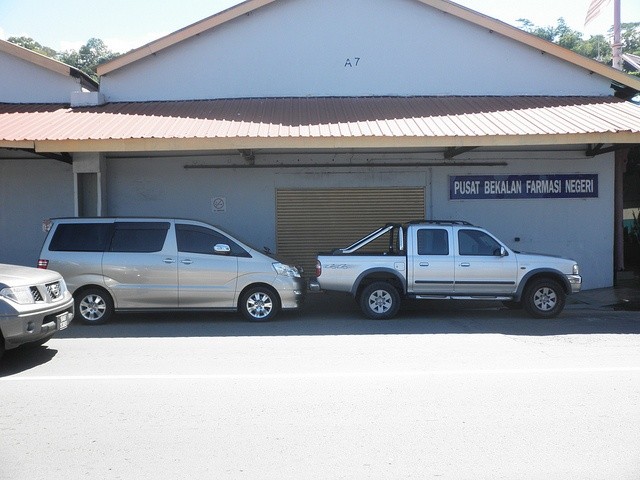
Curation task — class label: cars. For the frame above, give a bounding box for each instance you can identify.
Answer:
[0,263,76,359]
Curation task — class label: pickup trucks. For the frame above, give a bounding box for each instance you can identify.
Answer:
[315,217,582,319]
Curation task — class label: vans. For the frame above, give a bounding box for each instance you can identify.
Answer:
[38,216,307,325]
